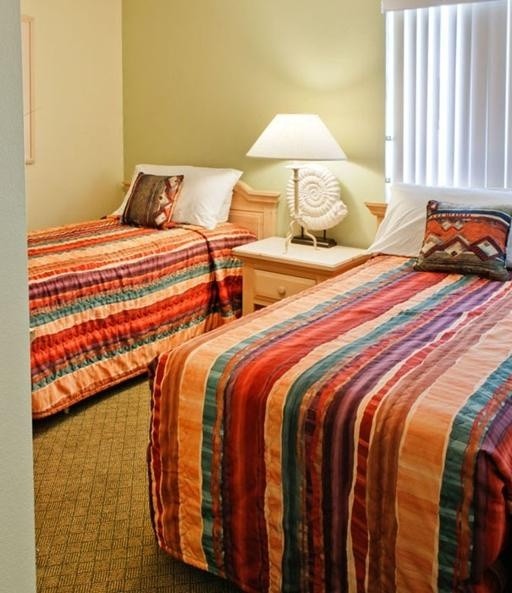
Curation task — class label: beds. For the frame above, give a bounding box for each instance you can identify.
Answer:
[146,200,511,593]
[28,180,282,423]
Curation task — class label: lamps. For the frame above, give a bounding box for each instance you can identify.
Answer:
[245,112,348,255]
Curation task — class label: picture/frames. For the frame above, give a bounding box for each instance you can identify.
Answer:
[21,14,34,167]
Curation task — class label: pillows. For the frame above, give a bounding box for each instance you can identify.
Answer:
[412,199,511,281]
[121,171,184,229]
[218,190,233,224]
[111,165,244,230]
[364,183,511,269]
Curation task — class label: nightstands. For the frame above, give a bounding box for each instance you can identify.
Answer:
[231,236,372,318]
